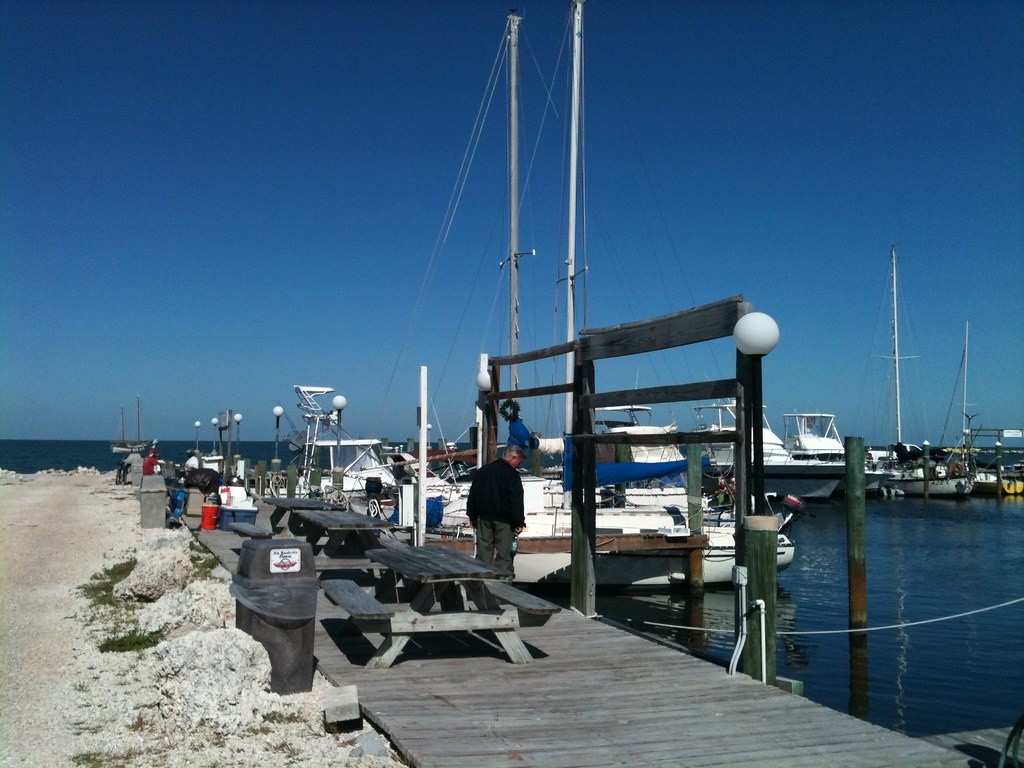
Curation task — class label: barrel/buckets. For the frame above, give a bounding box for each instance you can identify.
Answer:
[201,504,219,530]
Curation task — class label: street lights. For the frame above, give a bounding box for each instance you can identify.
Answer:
[329,393,348,495]
[195,421,201,454]
[211,417,219,457]
[271,406,286,474]
[234,414,243,477]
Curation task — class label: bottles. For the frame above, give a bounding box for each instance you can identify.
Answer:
[512,536,517,552]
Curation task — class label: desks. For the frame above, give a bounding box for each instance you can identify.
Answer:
[270,511,410,588]
[324,545,553,670]
[232,498,348,556]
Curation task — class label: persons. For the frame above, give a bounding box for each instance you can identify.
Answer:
[142,448,161,476]
[645,484,652,489]
[466,446,526,585]
[717,479,725,493]
[185,450,198,478]
[729,477,735,490]
[866,452,872,463]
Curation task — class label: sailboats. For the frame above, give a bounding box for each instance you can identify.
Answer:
[276,0,1024,595]
[111,392,155,455]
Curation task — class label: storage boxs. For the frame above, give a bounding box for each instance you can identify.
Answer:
[219,486,247,504]
[218,507,259,531]
[230,496,253,507]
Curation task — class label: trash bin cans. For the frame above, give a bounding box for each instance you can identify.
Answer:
[233,539,319,695]
[139,475,167,529]
[123,453,142,483]
[131,458,144,486]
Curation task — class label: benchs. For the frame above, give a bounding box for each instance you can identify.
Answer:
[483,579,561,615]
[227,521,272,536]
[323,579,395,619]
[378,539,408,549]
